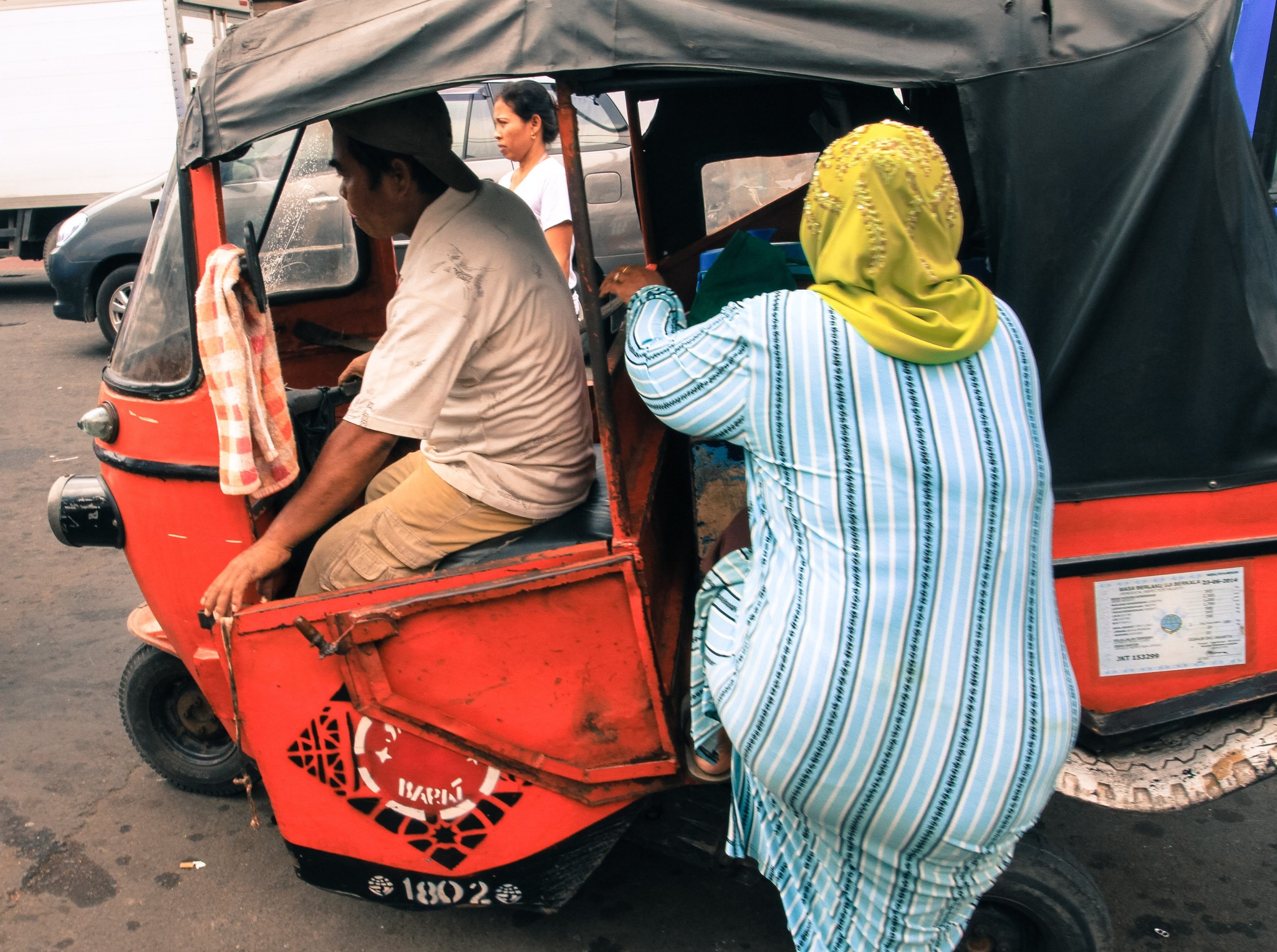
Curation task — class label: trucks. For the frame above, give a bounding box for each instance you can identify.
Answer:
[0,0,261,267]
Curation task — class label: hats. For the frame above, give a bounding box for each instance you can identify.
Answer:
[327,91,480,192]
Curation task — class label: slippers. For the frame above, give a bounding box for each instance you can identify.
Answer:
[681,691,730,782]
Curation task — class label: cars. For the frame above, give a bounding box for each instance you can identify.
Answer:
[43,84,649,357]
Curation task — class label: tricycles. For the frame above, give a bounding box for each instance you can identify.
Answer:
[51,0,1276,951]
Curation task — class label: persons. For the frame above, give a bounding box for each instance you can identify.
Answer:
[199,86,595,620]
[605,116,1087,951]
[491,74,590,323]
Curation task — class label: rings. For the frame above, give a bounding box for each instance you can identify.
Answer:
[612,272,621,281]
[622,265,631,272]
